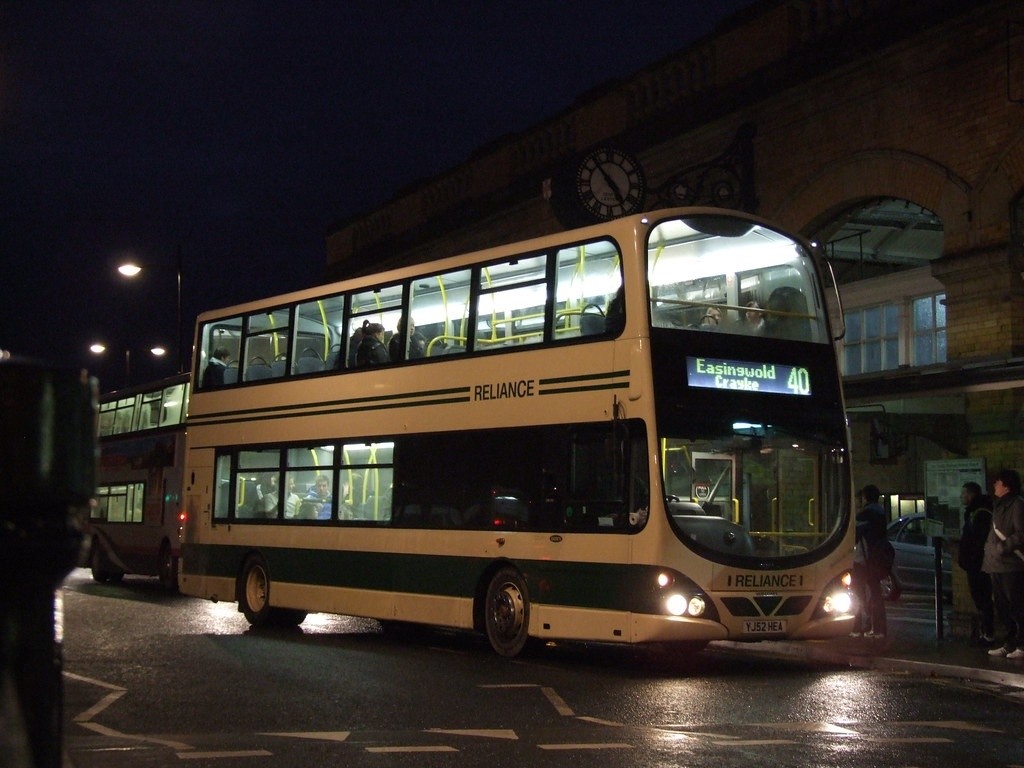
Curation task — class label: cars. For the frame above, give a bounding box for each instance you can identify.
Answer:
[880,511,956,604]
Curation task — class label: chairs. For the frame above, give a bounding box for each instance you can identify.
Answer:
[225,344,340,386]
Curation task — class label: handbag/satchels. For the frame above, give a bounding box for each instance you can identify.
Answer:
[867,539,895,578]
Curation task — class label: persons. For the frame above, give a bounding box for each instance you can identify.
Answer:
[982,469,1024,658]
[202,346,230,386]
[297,473,363,519]
[706,307,723,326]
[854,483,888,639]
[0,350,100,766]
[956,481,995,646]
[238,473,301,519]
[334,316,429,372]
[603,283,623,332]
[744,300,765,334]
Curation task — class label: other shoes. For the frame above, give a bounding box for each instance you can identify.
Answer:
[865,631,886,639]
[987,647,1010,657]
[848,631,862,642]
[1005,647,1024,659]
[969,636,995,649]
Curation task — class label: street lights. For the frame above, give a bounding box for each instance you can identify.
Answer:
[89,342,132,388]
[117,263,185,375]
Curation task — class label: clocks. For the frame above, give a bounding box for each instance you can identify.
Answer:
[578,145,644,217]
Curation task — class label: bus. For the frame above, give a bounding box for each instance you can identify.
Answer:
[180,203,864,664]
[83,431,184,597]
